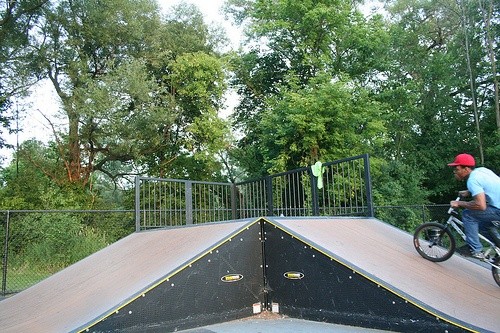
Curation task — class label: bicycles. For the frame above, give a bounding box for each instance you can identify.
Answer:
[413,190,500,286]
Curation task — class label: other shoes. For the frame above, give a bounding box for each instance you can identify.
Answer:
[455,243,481,257]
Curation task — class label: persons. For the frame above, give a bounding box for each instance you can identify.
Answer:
[446,154,500,257]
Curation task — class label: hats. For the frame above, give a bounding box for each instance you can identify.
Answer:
[447,152,476,166]
